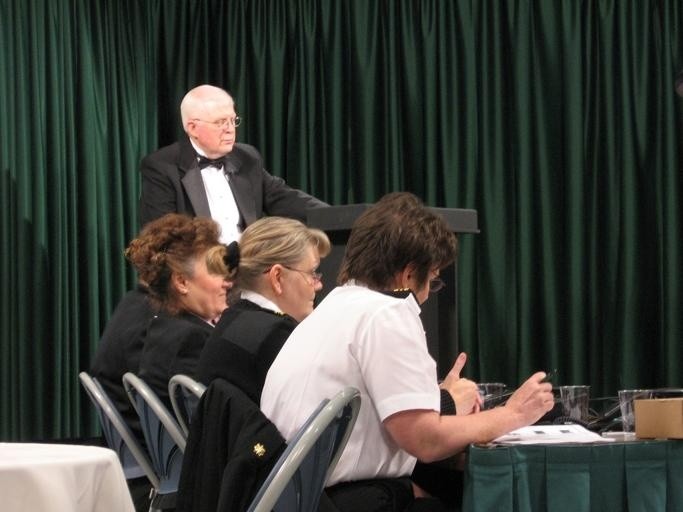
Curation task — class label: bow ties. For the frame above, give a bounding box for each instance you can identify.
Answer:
[198,155,227,169]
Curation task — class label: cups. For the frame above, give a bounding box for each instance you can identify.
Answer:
[560,385,590,420]
[619,390,654,432]
[477,384,504,410]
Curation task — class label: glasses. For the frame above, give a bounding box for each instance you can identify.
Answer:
[263,262,324,285]
[187,117,243,130]
[408,259,447,294]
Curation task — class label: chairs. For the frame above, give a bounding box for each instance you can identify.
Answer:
[168,373,209,444]
[242,387,364,512]
[79,370,161,492]
[123,372,189,512]
[203,375,288,512]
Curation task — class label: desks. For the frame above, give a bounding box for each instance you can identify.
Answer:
[308,202,480,379]
[0,440,137,512]
[449,437,683,512]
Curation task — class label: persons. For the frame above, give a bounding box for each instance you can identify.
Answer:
[140,238,230,511]
[94,214,221,511]
[139,84,333,246]
[261,192,555,511]
[195,216,330,411]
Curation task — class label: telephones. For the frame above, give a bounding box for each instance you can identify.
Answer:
[481,385,590,424]
[475,383,514,409]
[553,387,683,435]
